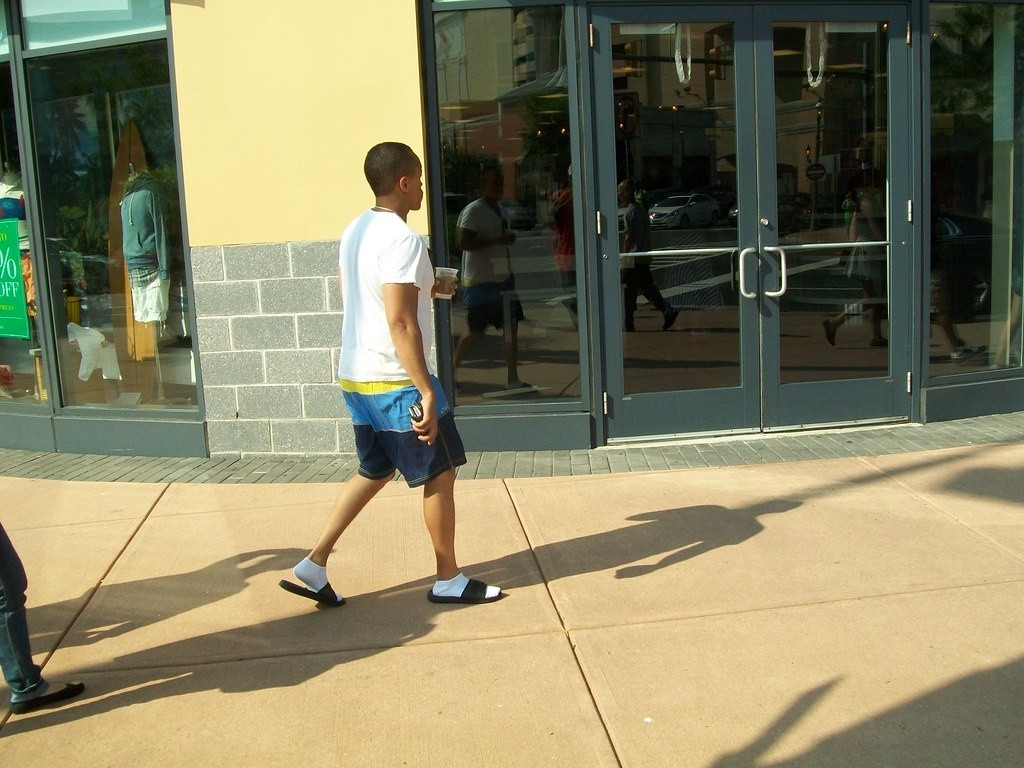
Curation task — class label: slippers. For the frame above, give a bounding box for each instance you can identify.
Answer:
[504,383,538,392]
[427,579,502,604]
[279,580,346,608]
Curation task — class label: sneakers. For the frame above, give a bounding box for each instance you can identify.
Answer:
[951,344,989,364]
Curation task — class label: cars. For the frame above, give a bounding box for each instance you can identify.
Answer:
[728,190,844,239]
[497,201,536,231]
[927,208,991,325]
[648,192,723,229]
[617,200,630,231]
[48,237,110,297]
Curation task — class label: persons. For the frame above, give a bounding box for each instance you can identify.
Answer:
[120,166,169,323]
[0,163,41,319]
[926,272,987,365]
[450,158,534,392]
[545,155,580,331]
[822,185,891,348]
[278,140,503,605]
[615,182,680,332]
[0,528,88,716]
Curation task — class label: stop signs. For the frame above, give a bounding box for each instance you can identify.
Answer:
[806,163,825,182]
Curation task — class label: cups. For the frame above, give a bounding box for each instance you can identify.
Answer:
[432,267,459,300]
[503,229,515,245]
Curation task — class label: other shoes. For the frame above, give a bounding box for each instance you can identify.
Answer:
[870,336,888,348]
[662,308,679,331]
[9,680,84,714]
[622,325,635,332]
[823,319,836,346]
